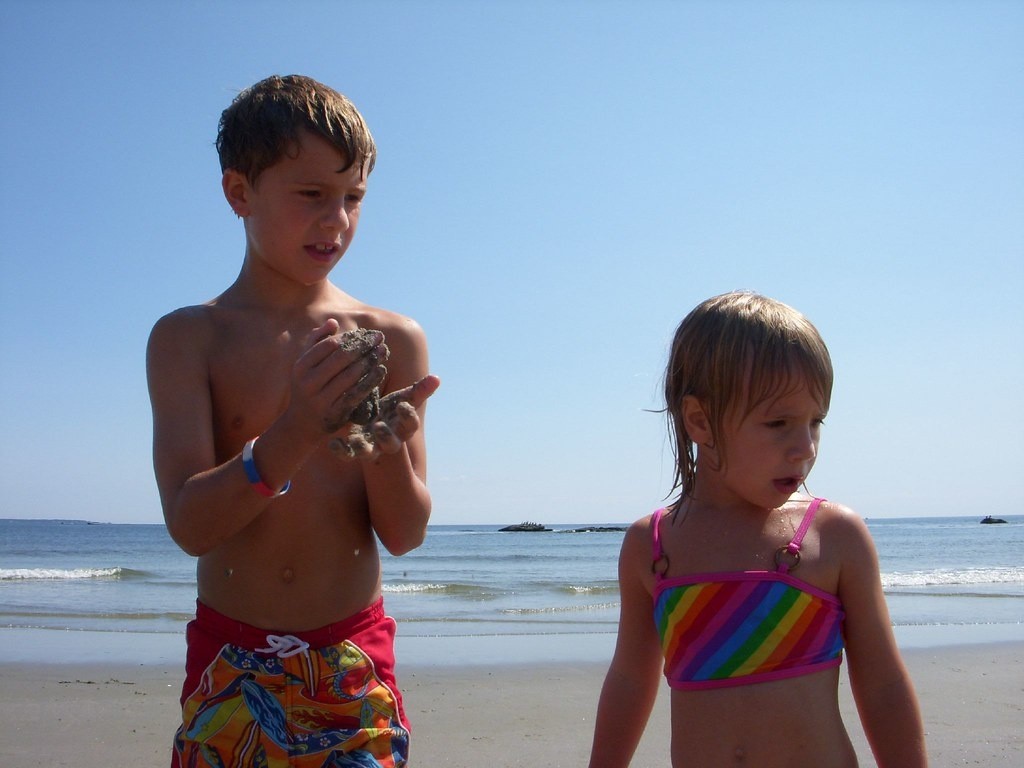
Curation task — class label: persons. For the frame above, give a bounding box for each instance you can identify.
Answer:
[588,290,930,768]
[145,73,440,768]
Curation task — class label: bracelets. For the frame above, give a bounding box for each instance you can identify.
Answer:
[243,436,291,497]
